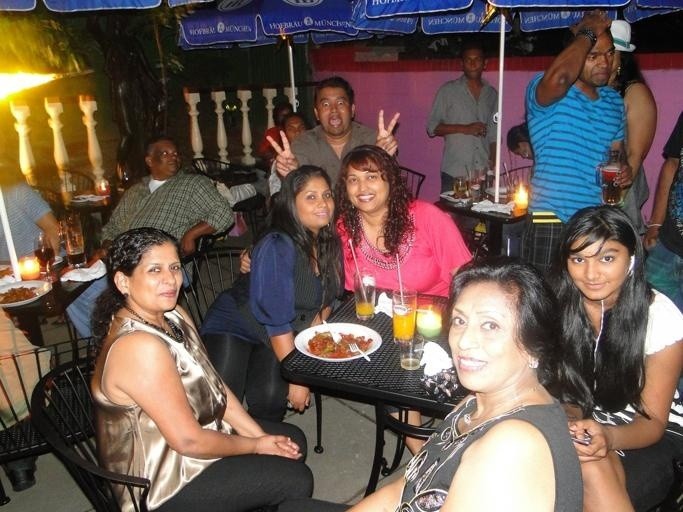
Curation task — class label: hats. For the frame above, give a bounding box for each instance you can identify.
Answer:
[609,19,637,53]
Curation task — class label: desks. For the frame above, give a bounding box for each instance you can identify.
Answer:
[277,284,473,499]
[432,180,537,269]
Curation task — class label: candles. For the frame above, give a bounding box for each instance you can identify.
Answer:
[414,302,444,340]
[511,182,531,211]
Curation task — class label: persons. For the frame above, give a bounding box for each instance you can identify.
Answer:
[550,207,683,512]
[202,48,499,451]
[507,10,681,306]
[1,138,234,338]
[350,252,583,511]
[90,226,315,512]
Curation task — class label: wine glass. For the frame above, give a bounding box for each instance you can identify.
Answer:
[455,177,466,208]
[35,233,55,282]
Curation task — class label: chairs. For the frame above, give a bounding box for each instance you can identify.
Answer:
[0,334,101,494]
[167,245,255,338]
[173,236,210,299]
[28,354,151,512]
[48,164,95,194]
[224,185,267,243]
[28,185,63,215]
[190,157,242,184]
[489,165,533,190]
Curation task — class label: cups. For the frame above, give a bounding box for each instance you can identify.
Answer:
[60,215,85,266]
[469,169,480,202]
[393,290,417,342]
[354,270,376,320]
[398,334,422,371]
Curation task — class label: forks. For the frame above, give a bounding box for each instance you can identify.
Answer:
[349,344,371,362]
[323,320,341,343]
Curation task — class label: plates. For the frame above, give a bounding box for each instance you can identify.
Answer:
[0,280,52,308]
[71,195,89,202]
[295,322,382,362]
[485,187,506,198]
[440,190,481,202]
[53,256,63,266]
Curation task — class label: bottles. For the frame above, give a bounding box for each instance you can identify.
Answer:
[597,151,622,205]
